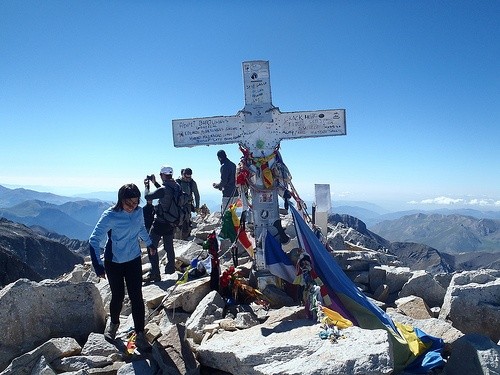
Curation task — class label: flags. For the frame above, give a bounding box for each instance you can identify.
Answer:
[291,204,446,375]
[127,155,354,353]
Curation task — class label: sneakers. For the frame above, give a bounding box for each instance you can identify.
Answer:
[135,333,152,353]
[104,322,120,342]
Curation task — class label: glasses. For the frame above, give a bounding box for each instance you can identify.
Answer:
[184,175,191,178]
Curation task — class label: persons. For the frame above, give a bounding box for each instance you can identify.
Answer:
[142,199,156,234]
[89,183,157,353]
[140,166,183,281]
[175,167,200,241]
[213,149,239,218]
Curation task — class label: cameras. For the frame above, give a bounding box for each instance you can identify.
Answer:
[147,175,152,180]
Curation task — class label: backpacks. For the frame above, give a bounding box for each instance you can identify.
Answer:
[160,182,193,227]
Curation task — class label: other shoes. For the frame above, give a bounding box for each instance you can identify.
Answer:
[165,267,175,274]
[142,275,160,281]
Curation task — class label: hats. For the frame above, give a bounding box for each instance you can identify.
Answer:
[160,166,174,174]
